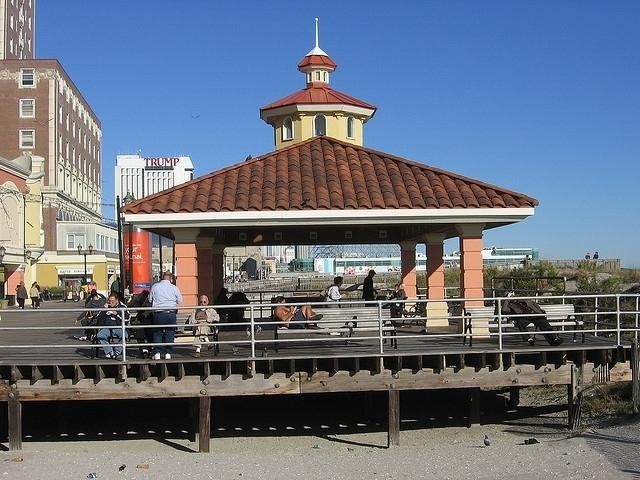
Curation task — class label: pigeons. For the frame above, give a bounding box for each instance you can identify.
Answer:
[245,155,252,161]
[255,324,261,335]
[232,343,240,354]
[246,328,251,337]
[262,346,268,357]
[484,435,490,446]
[348,322,355,334]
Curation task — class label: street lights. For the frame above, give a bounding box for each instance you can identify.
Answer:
[77,243,94,276]
[222,251,227,283]
[123,191,138,294]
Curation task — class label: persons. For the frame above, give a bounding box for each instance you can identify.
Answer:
[274,296,323,329]
[386,283,405,324]
[362,270,378,307]
[189,295,220,358]
[323,277,345,308]
[502,291,563,345]
[15,281,40,309]
[73,271,183,360]
[214,288,240,322]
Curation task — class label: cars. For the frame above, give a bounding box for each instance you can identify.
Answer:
[224,274,254,283]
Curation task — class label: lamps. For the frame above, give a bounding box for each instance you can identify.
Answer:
[26,249,36,260]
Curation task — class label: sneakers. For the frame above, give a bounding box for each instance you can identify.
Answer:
[310,313,324,320]
[550,336,564,347]
[151,352,161,361]
[105,351,123,361]
[194,347,202,356]
[206,341,215,350]
[529,339,537,347]
[164,353,171,360]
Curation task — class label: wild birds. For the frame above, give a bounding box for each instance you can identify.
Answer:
[191,115,200,119]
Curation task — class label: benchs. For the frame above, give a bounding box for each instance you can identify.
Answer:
[89,310,223,357]
[271,305,402,353]
[460,303,587,347]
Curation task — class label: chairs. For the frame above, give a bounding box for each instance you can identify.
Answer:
[375,288,427,328]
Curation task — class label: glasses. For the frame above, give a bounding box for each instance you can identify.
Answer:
[200,301,207,304]
